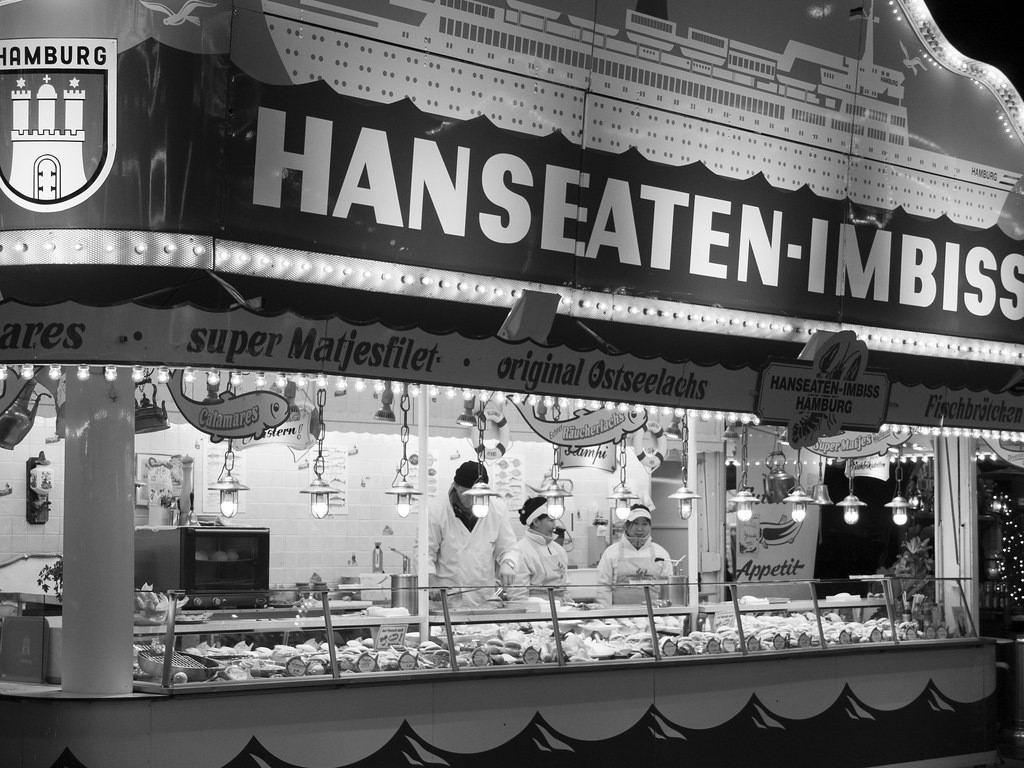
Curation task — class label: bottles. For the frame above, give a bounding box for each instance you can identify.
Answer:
[373,541,384,574]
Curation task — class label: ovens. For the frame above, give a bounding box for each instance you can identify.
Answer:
[135,524,272,610]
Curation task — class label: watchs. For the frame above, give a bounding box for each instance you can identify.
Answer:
[502,559,516,569]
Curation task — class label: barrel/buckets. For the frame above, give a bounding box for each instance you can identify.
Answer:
[390,573,419,616]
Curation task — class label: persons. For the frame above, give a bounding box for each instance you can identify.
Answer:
[503,496,577,609]
[596,503,677,608]
[411,459,521,639]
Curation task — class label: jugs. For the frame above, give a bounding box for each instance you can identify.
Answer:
[0,377,55,450]
[760,450,795,504]
[134,377,171,435]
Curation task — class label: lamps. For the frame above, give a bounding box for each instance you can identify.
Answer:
[208,439,249,517]
[783,449,815,522]
[0,364,761,425]
[667,413,701,520]
[385,394,422,517]
[728,424,760,522]
[462,402,499,518]
[539,443,573,520]
[606,434,640,520]
[300,389,338,519]
[884,455,916,525]
[836,459,867,525]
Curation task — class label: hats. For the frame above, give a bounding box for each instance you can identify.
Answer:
[526,501,548,526]
[625,509,651,522]
[454,460,490,488]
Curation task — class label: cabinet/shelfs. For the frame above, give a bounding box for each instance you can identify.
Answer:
[134,576,980,693]
